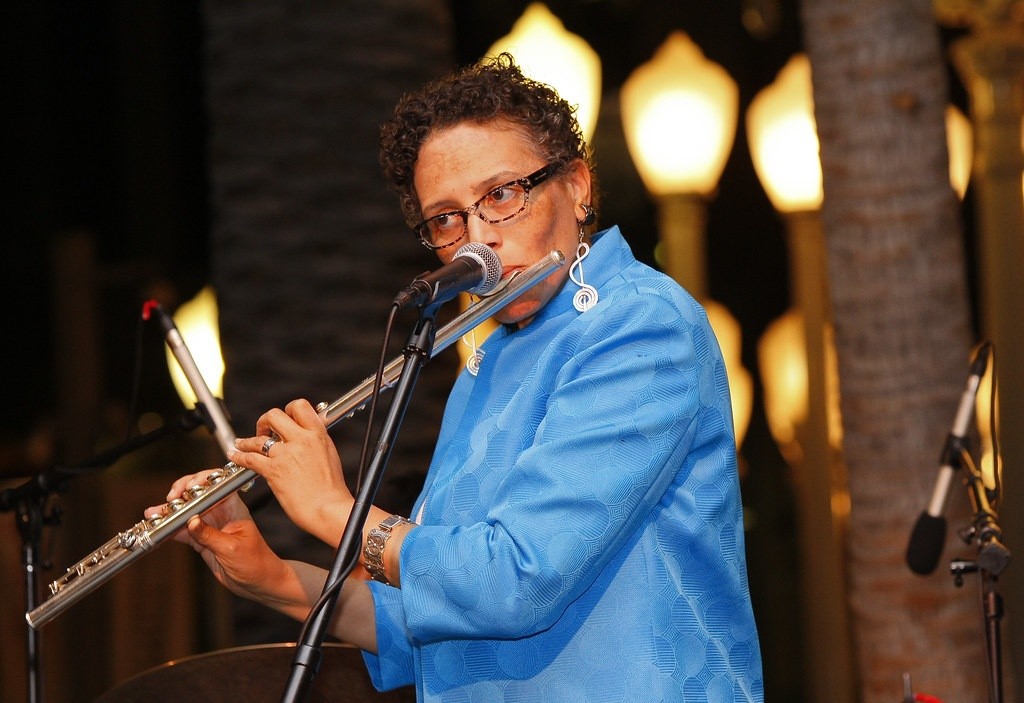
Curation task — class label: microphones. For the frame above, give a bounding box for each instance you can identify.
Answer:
[157,306,254,493]
[392,243,503,314]
[905,343,991,576]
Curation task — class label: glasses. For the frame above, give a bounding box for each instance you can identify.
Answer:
[412,155,573,253]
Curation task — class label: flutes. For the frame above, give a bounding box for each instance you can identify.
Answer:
[23,249,567,630]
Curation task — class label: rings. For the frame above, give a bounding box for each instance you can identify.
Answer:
[262,438,276,456]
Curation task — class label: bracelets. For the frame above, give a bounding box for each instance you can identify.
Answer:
[363,515,419,588]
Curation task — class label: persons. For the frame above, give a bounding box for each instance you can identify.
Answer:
[145,55,764,703]
